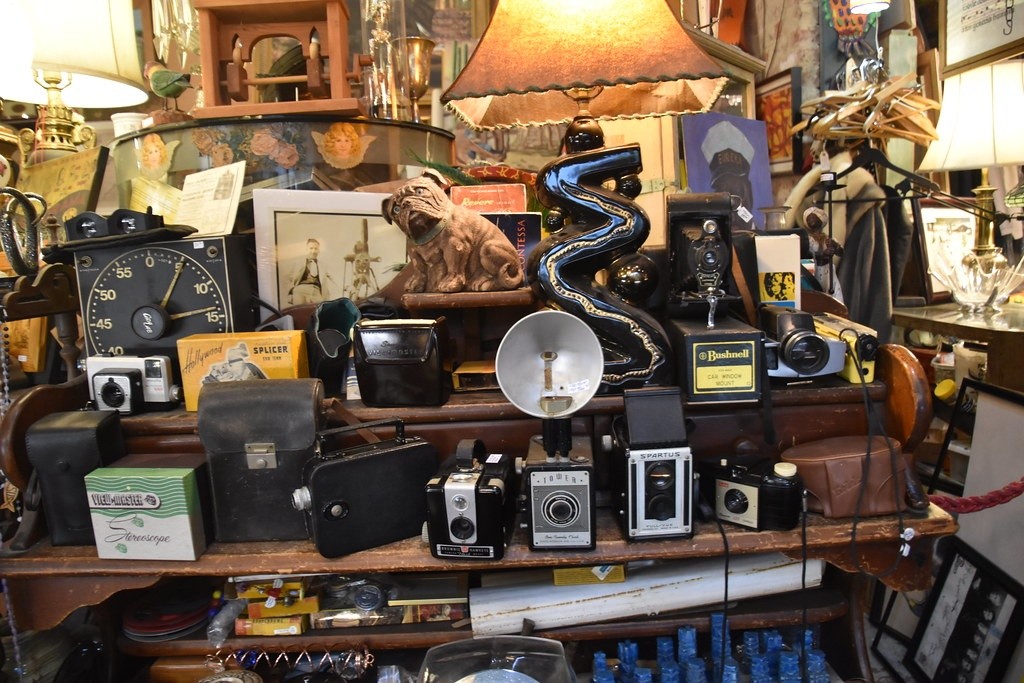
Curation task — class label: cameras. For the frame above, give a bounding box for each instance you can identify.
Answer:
[76,352,183,417]
[422,192,883,560]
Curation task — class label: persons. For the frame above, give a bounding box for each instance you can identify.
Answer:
[285,239,338,306]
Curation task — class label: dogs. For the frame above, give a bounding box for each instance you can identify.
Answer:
[382,168,525,293]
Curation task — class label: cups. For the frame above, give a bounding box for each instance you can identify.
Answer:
[110,112,148,138]
[931,357,954,385]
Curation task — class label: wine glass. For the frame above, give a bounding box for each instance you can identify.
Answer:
[392,36,436,124]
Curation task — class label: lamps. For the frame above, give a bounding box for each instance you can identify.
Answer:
[850,0,892,14]
[442,0,731,397]
[918,57,1024,305]
[0,0,149,167]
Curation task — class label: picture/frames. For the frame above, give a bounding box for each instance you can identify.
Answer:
[252,187,407,324]
[912,48,950,198]
[915,197,979,305]
[596,114,679,251]
[84,1,159,121]
[938,0,1024,80]
[754,67,802,175]
[252,0,492,108]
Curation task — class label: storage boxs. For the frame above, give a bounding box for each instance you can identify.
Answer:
[177,330,309,412]
[84,468,207,561]
[755,233,801,309]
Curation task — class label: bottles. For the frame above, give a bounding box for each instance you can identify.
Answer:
[934,378,977,413]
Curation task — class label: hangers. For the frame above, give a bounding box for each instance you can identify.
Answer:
[781,59,943,229]
